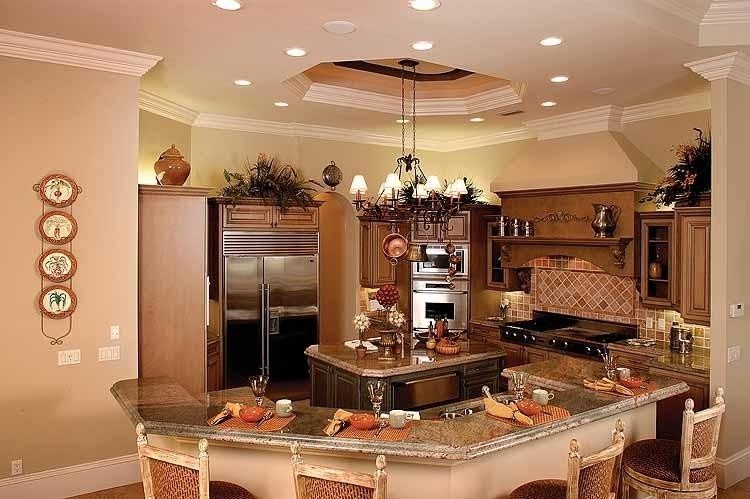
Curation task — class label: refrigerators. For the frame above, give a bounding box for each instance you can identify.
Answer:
[221,228,320,402]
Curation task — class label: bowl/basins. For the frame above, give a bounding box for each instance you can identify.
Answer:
[414,341,429,350]
[348,413,375,430]
[620,377,644,388]
[415,331,460,343]
[426,342,436,350]
[238,407,264,422]
[516,401,542,416]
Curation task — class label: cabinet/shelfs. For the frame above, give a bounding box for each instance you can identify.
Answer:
[501,344,547,389]
[672,206,711,327]
[356,215,396,289]
[207,339,221,392]
[487,221,521,292]
[639,212,674,310]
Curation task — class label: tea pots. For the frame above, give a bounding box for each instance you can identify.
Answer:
[590,203,619,239]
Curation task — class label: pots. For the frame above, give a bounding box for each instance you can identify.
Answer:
[381,227,431,266]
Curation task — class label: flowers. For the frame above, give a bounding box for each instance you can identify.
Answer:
[375,284,400,330]
[352,312,372,346]
[389,311,406,328]
[637,115,711,208]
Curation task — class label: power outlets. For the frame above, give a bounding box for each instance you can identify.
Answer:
[10,459,24,474]
[58,349,81,365]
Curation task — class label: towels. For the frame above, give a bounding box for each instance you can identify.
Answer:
[211,401,242,424]
[482,398,533,427]
[585,376,634,397]
[324,408,353,436]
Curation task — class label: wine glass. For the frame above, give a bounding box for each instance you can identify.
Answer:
[364,380,387,418]
[248,374,271,406]
[509,372,531,401]
[601,352,618,381]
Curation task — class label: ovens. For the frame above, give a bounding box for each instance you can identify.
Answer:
[411,243,471,336]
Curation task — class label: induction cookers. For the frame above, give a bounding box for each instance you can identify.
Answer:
[498,319,634,359]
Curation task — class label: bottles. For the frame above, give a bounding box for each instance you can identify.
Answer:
[679,328,693,356]
[435,317,443,336]
[495,215,535,237]
[669,321,680,349]
[442,317,448,334]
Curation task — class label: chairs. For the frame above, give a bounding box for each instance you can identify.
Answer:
[621,387,726,499]
[289,439,387,499]
[508,418,626,498]
[135,422,256,499]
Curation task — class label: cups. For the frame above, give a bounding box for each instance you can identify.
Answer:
[389,410,414,428]
[649,263,662,278]
[276,399,294,419]
[617,367,631,380]
[532,389,554,406]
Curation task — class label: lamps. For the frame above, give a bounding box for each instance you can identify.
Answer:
[348,58,468,234]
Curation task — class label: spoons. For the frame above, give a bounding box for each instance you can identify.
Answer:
[254,412,273,428]
[374,420,388,438]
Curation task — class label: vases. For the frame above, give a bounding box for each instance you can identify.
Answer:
[377,330,398,361]
[355,347,367,358]
